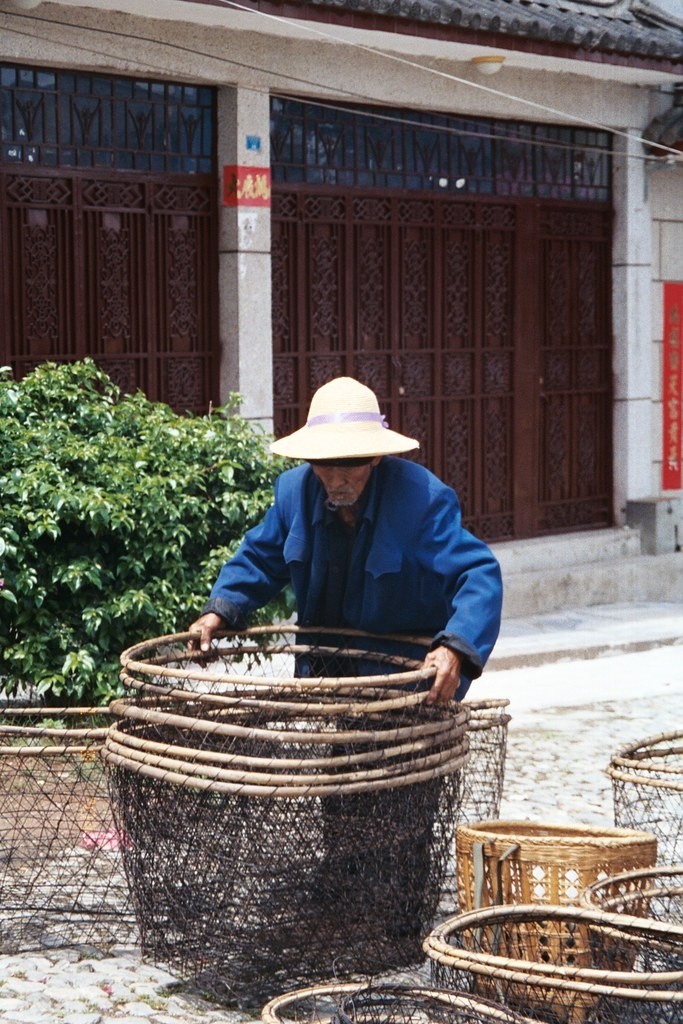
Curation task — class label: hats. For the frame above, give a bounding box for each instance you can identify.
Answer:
[270,377,420,460]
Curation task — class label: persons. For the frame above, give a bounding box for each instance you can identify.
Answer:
[190,377,503,939]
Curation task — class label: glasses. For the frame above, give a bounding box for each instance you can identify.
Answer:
[304,456,375,468]
